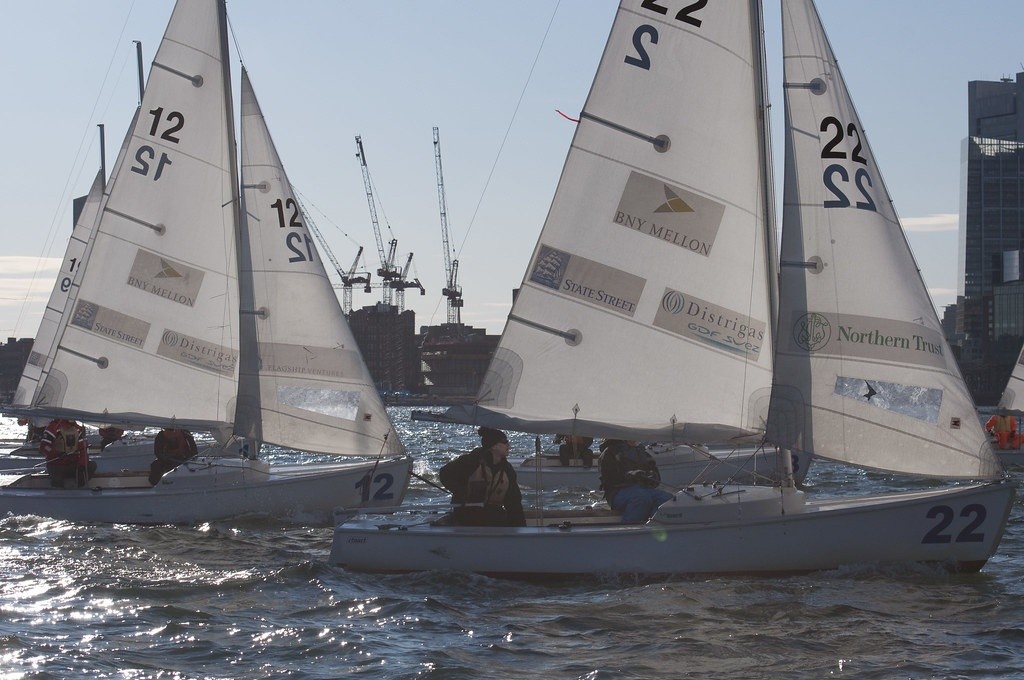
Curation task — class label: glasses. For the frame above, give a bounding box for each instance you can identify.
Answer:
[499,439,509,445]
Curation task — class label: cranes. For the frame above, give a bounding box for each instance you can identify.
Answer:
[287,178,372,330]
[429,125,465,354]
[353,133,428,393]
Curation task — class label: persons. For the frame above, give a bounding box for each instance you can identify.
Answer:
[986,415,1016,438]
[98,427,124,452]
[439,427,527,527]
[40,418,97,488]
[554,433,593,466]
[148,429,197,486]
[598,439,672,523]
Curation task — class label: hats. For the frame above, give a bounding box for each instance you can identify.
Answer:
[478,427,507,449]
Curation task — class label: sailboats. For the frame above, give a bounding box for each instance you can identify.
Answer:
[315,1,1013,593]
[0,0,413,531]
[987,335,1024,468]
